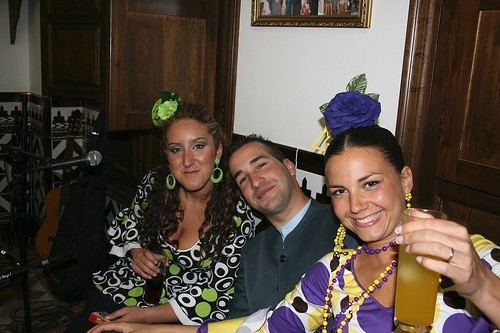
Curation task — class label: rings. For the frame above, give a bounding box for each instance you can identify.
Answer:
[447,247,454,261]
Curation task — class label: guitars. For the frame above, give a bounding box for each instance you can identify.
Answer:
[34,109,84,260]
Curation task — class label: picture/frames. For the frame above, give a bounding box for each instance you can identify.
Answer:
[250,0,374,29]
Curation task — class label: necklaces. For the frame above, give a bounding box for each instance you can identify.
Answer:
[323,240,398,333]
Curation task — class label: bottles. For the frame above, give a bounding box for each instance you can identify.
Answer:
[82,112,96,126]
[64,123,69,128]
[53,122,62,129]
[18,117,23,124]
[27,116,31,129]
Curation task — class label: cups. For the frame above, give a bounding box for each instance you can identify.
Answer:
[393,207,448,333]
[143,250,167,303]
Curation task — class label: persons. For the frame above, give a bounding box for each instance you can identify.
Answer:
[64,101,255,333]
[260,0,360,16]
[223,134,360,333]
[87,125,500,333]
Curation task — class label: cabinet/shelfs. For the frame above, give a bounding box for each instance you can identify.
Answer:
[40,0,240,145]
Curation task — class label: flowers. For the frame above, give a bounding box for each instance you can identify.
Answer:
[150,91,183,127]
[319,73,382,135]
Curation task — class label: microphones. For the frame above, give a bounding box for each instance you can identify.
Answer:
[30,151,103,171]
